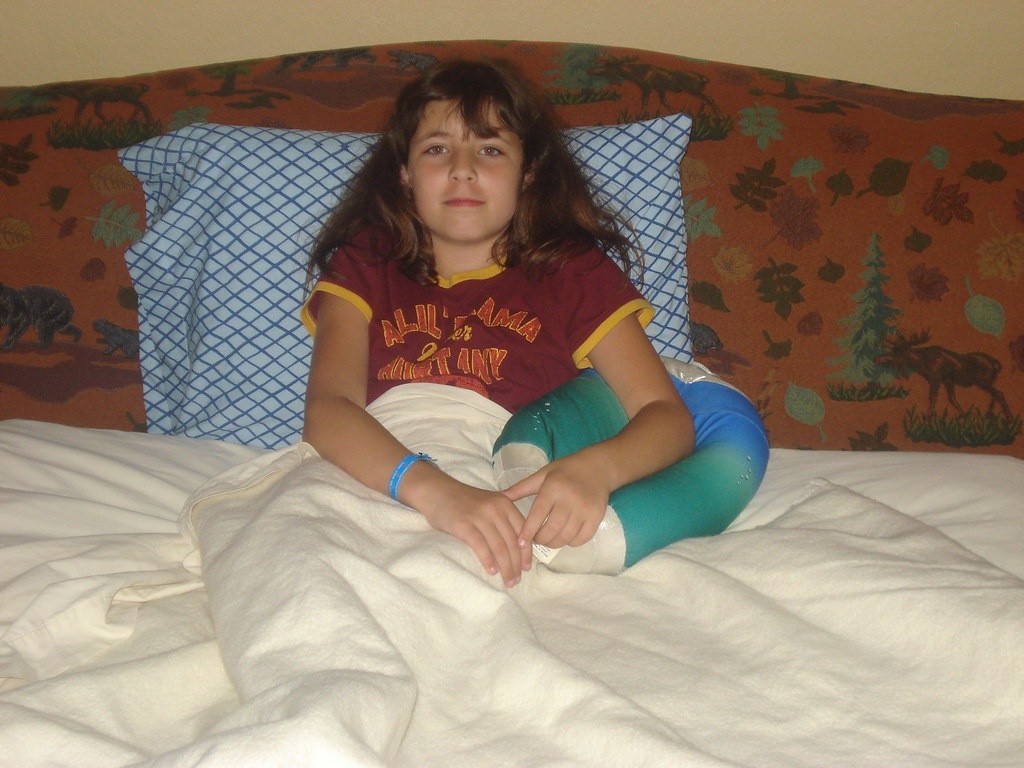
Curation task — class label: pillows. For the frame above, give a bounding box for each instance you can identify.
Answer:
[117,111,695,453]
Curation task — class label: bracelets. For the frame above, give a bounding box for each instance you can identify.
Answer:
[389,453,435,500]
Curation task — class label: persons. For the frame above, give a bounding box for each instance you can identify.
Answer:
[301,61,695,768]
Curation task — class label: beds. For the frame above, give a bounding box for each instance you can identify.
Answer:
[0,38,1024,768]
